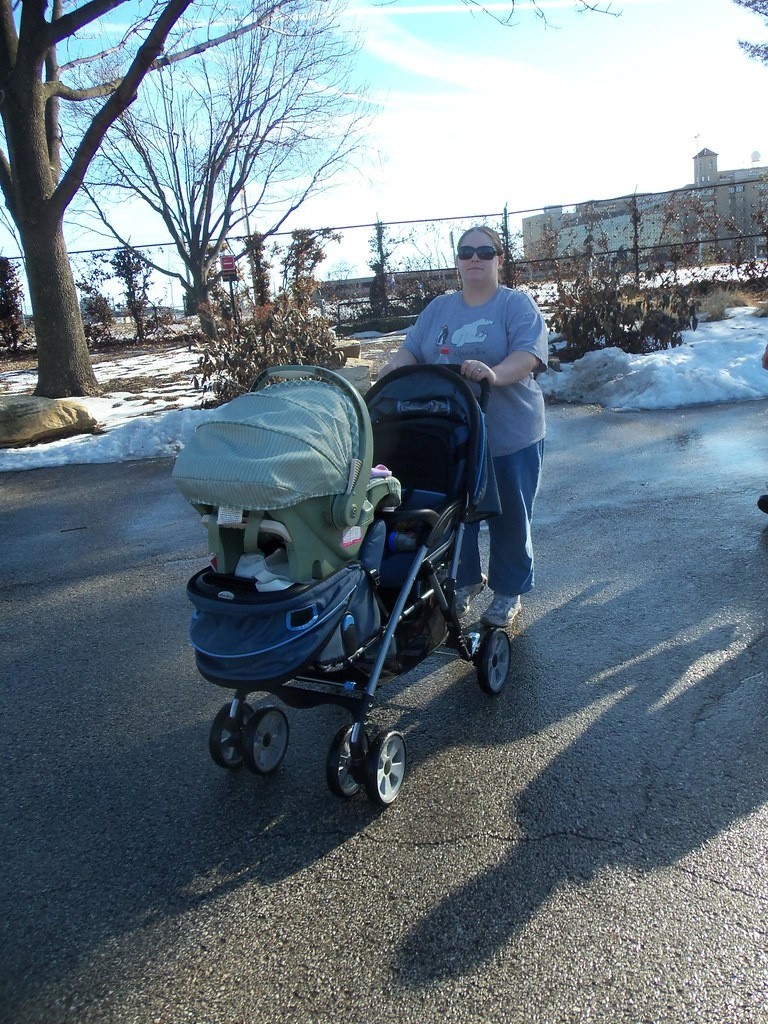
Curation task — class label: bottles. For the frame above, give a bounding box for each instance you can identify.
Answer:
[435,348,450,364]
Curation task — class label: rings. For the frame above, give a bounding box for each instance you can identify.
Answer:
[478,369,481,371]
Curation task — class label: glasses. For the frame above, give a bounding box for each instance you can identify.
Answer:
[457,246,499,260]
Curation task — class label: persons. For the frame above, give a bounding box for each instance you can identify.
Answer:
[379,226,550,629]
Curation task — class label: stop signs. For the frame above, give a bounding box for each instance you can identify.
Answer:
[221,256,235,270]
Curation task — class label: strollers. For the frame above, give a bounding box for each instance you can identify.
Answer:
[170,363,513,809]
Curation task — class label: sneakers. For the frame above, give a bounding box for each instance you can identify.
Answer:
[480,592,522,627]
[456,573,488,618]
